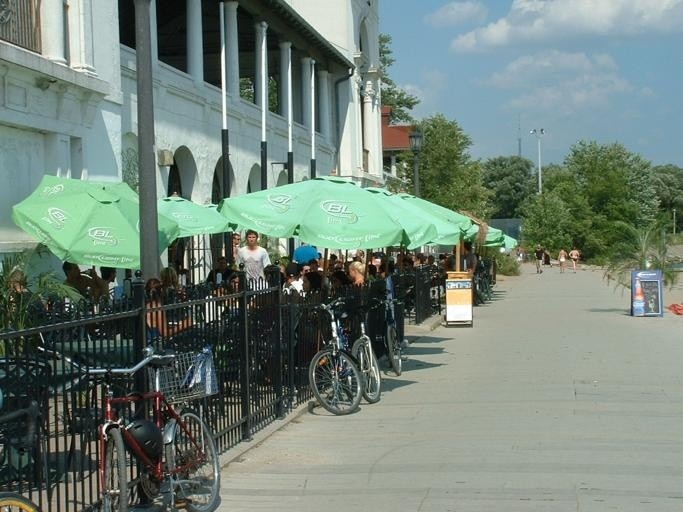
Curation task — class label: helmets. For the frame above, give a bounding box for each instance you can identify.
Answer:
[123,421,164,458]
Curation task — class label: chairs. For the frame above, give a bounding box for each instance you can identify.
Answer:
[0,266,443,490]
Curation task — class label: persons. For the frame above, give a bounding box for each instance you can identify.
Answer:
[542,247,552,267]
[326,240,485,301]
[568,246,579,273]
[233,233,240,258]
[533,243,546,275]
[557,248,568,273]
[14,259,324,356]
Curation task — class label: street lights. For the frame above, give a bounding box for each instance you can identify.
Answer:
[530,128,546,194]
[672,209,677,234]
[408,132,424,197]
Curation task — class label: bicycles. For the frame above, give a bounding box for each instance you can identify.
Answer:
[308,289,403,415]
[38,345,221,511]
[473,274,491,303]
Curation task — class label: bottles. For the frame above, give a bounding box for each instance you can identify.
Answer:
[635,278,641,297]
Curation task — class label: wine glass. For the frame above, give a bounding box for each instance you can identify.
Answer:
[649,299,655,313]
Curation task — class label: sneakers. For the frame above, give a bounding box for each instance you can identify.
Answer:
[537,269,543,274]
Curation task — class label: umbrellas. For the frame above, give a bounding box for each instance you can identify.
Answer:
[363,180,520,285]
[153,189,234,268]
[216,167,411,301]
[10,172,181,294]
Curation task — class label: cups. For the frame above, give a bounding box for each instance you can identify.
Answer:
[217,273,222,284]
